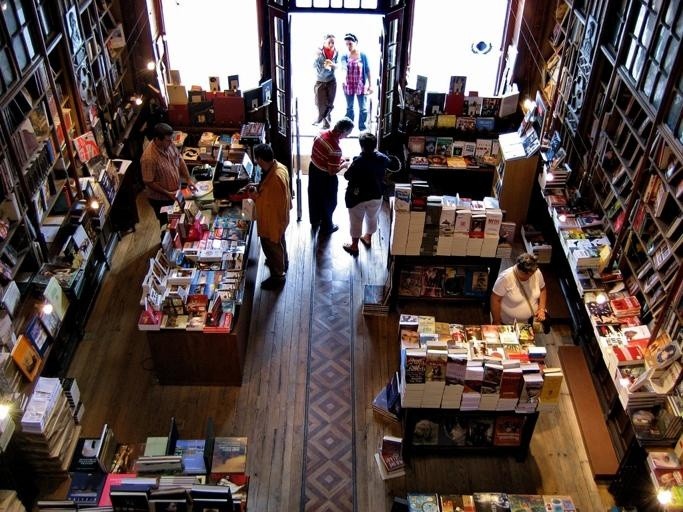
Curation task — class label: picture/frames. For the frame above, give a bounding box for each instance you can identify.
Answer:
[579,14,601,64]
[64,3,84,55]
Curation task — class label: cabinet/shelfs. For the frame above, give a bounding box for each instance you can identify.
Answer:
[0,0,147,382]
[392,255,501,313]
[407,153,494,200]
[520,1,683,511]
[400,408,539,464]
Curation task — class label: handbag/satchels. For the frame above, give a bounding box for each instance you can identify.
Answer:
[532,316,544,334]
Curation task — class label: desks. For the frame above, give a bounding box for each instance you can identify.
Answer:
[146,164,261,388]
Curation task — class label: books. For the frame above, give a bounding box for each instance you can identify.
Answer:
[363,0,682,512]
[0,1,252,512]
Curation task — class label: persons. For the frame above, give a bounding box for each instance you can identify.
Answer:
[308,117,354,234]
[344,133,391,255]
[246,143,292,287]
[341,33,374,136]
[314,34,339,129]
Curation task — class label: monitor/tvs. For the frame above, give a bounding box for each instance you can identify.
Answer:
[241,152,254,178]
[212,145,224,181]
